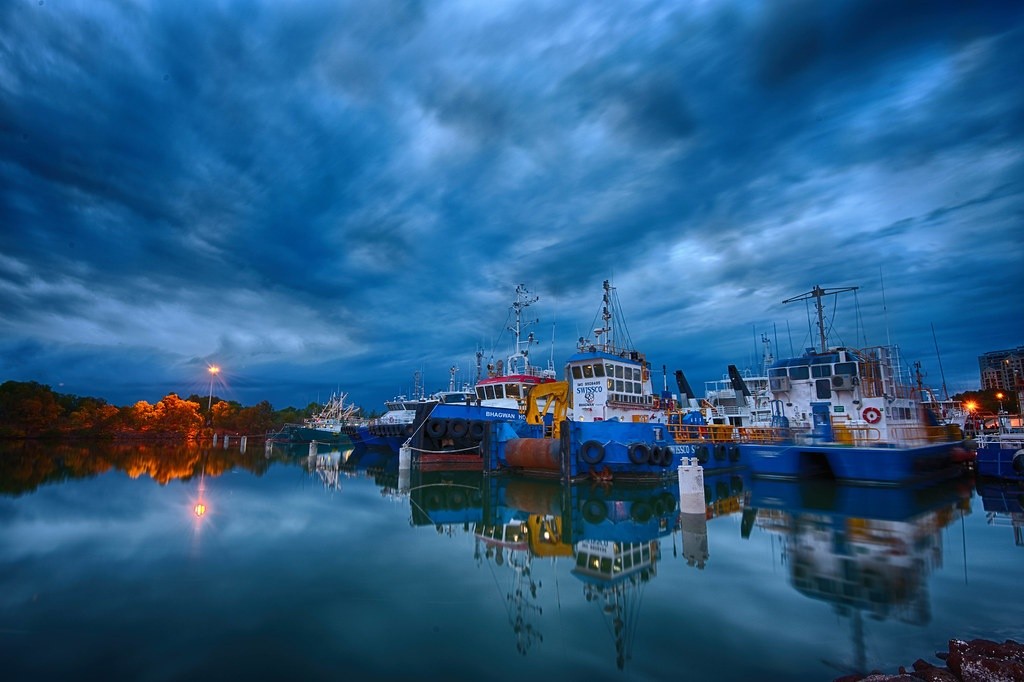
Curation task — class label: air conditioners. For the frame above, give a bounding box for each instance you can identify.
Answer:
[770,376,791,392]
[831,374,852,390]
[644,395,653,405]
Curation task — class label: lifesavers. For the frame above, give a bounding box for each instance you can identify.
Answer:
[730,446,741,462]
[628,443,650,465]
[368,424,401,438]
[340,426,357,434]
[469,419,486,439]
[862,406,881,424]
[579,440,606,466]
[448,417,468,438]
[715,445,727,461]
[701,400,709,408]
[731,473,744,496]
[629,492,676,523]
[717,480,730,501]
[581,498,608,525]
[426,417,447,439]
[650,446,662,464]
[705,485,712,504]
[696,445,710,463]
[425,487,483,512]
[661,446,673,468]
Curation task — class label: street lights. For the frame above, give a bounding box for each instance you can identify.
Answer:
[206,365,221,412]
[194,461,209,518]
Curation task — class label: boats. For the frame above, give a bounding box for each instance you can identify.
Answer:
[265,387,379,455]
[367,266,753,481]
[363,462,741,675]
[737,487,1023,675]
[705,263,1023,488]
